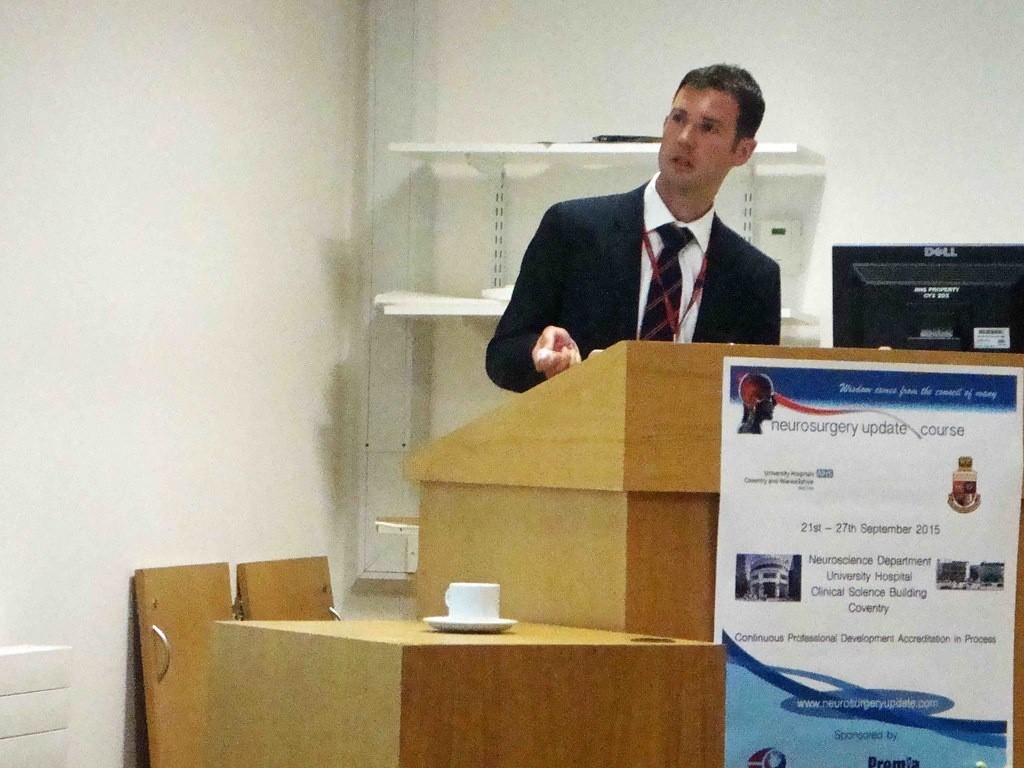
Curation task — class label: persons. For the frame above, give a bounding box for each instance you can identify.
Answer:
[485,63,781,394]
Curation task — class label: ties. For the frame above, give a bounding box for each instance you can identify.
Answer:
[641,222,694,344]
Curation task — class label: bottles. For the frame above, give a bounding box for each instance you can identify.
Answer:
[952,456,976,506]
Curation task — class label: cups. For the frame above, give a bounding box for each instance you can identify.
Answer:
[445,582,500,622]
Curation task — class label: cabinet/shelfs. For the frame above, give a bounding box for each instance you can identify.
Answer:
[208,620,726,767]
[381,139,824,325]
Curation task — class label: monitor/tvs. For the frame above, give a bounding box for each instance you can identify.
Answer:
[831,245,1024,356]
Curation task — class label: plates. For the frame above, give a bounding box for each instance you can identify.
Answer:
[423,616,519,633]
